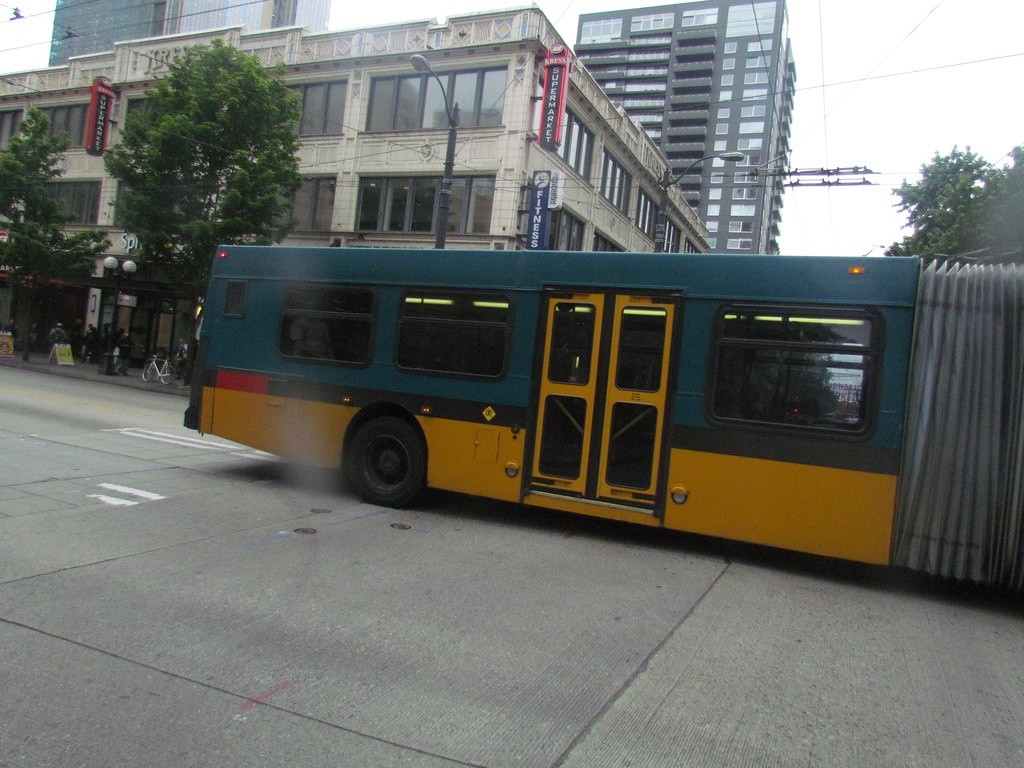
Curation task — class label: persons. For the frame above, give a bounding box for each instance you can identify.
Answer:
[67,319,111,365]
[2,319,16,335]
[289,300,332,358]
[177,337,188,380]
[114,329,136,375]
[48,323,66,348]
[30,322,45,348]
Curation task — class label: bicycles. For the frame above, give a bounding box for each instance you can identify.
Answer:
[141,353,177,385]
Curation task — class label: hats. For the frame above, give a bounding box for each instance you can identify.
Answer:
[57,323,63,327]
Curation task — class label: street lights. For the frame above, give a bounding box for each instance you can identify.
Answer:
[652,149,746,254]
[98,256,137,376]
[409,53,460,250]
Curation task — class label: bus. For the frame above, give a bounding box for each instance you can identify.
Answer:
[180,239,1024,600]
